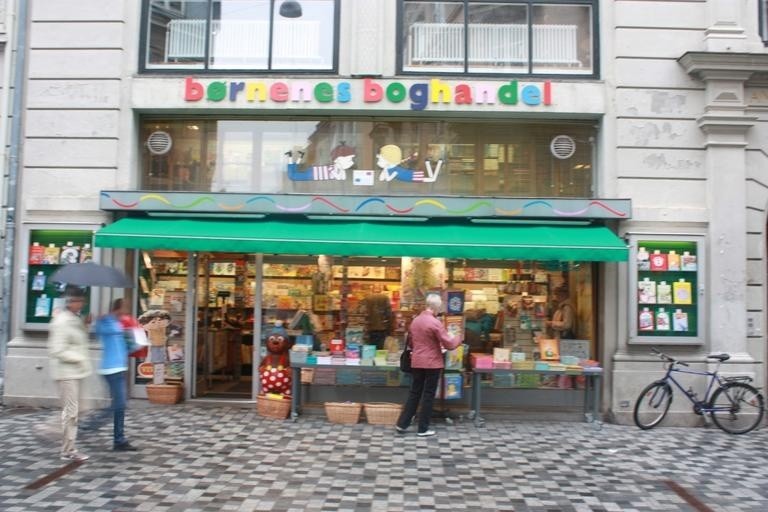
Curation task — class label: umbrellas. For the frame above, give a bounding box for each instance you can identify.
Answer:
[47,262,135,323]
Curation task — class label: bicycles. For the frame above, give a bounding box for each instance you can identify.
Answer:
[633,346,765,434]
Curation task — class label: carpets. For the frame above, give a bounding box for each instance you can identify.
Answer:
[228,379,253,393]
[203,390,252,398]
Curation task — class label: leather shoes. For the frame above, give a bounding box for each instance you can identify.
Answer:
[114,440,138,451]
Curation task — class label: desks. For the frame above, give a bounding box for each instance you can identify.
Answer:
[468,365,606,432]
[288,356,463,423]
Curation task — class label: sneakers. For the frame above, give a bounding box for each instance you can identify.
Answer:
[416,429,435,437]
[395,425,415,433]
[61,448,89,460]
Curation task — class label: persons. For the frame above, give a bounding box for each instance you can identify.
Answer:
[545,285,579,340]
[84,297,141,452]
[31,290,95,463]
[394,293,465,437]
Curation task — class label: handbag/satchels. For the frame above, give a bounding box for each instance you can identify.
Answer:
[399,328,413,374]
[124,317,147,358]
[559,328,575,339]
